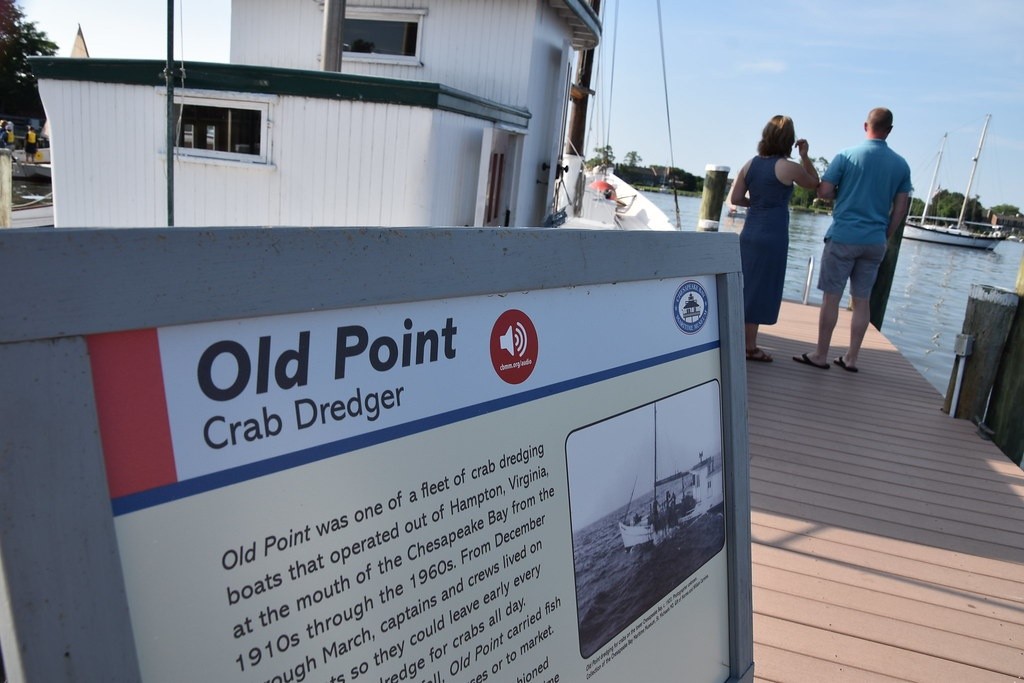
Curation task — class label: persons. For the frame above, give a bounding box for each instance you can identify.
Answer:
[23,126,38,163]
[725,115,820,363]
[0,119,16,163]
[792,107,913,373]
[975,209,983,222]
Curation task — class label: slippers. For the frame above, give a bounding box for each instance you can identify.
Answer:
[833,356,857,371]
[793,353,830,369]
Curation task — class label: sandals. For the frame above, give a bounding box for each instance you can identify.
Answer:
[746,347,773,362]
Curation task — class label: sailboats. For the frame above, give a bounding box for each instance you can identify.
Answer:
[618,402,723,547]
[13,22,91,180]
[902,111,1011,249]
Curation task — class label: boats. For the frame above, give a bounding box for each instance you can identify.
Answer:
[26,0,682,226]
